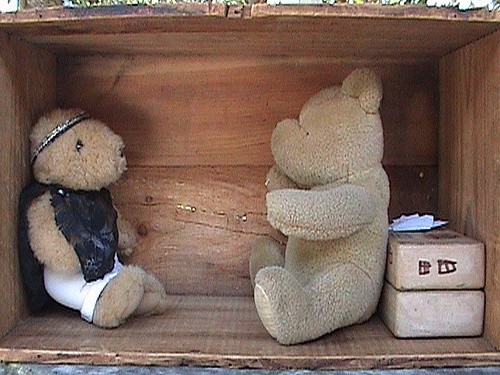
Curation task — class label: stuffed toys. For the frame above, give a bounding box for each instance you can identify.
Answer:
[18,108,169,328]
[250,67,391,344]
